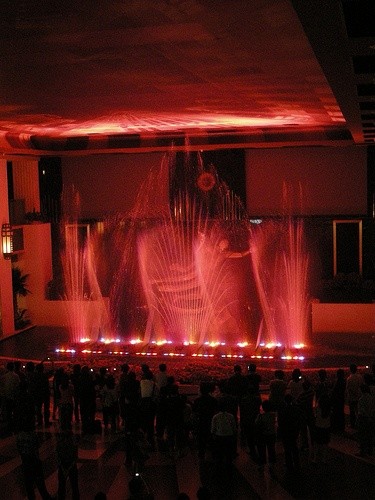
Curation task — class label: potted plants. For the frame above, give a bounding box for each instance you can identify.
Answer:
[311,272,375,334]
[41,280,111,328]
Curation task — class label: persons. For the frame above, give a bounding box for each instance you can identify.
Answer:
[217,363,289,426]
[13,424,57,499]
[0,363,193,472]
[296,382,314,445]
[347,363,363,427]
[356,385,374,458]
[256,400,278,481]
[209,401,238,471]
[92,473,213,500]
[313,370,332,429]
[54,417,82,499]
[310,401,331,465]
[280,394,303,469]
[286,368,306,406]
[360,360,375,422]
[190,383,219,471]
[328,368,345,434]
[238,384,261,466]
